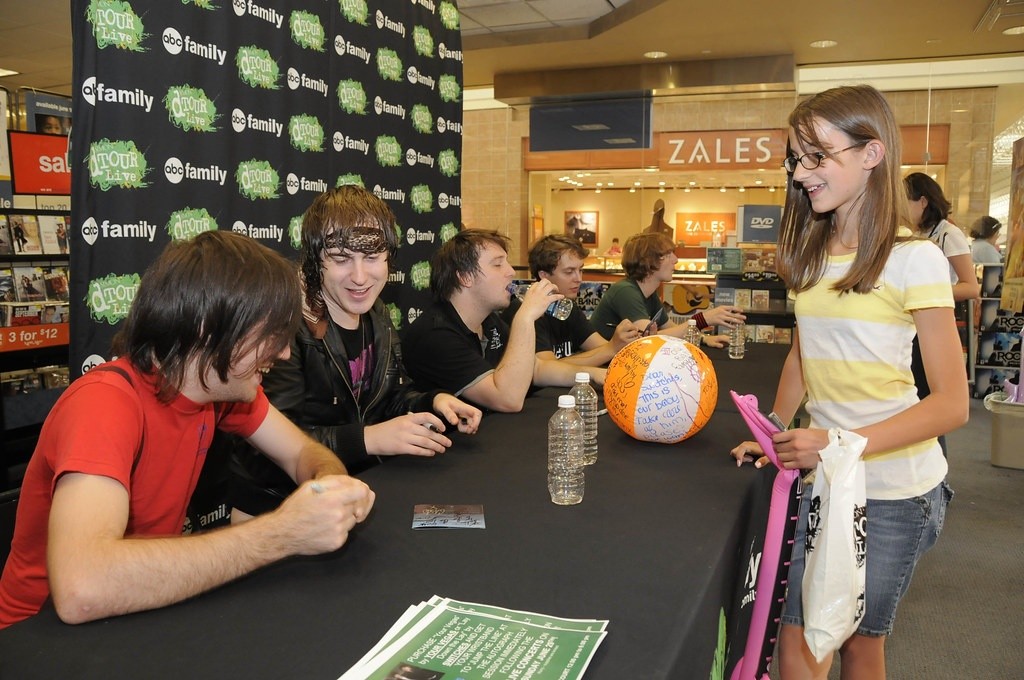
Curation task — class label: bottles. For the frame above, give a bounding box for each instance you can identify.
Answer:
[506,283,573,320]
[684,320,700,349]
[729,312,745,359]
[548,395,584,506]
[568,372,597,465]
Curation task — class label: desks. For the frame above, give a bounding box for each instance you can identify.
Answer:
[1,340,802,680]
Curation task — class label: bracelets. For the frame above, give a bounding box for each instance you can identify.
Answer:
[701,334,710,342]
[692,313,708,331]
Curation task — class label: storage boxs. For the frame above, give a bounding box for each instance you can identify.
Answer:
[984,392,1023,469]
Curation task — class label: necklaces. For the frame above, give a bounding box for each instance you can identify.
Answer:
[355,315,365,402]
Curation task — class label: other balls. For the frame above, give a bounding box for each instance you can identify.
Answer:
[603,334,719,444]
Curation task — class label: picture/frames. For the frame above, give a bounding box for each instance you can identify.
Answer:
[564,209,599,247]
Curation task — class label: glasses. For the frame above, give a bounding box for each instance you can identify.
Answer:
[782,141,865,172]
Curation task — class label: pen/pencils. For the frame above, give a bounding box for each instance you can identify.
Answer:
[769,413,787,432]
[597,408,608,417]
[405,410,440,433]
[606,323,643,333]
[311,482,324,492]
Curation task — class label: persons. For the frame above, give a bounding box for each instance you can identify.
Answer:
[904,174,979,461]
[18,224,27,251]
[399,229,607,413]
[21,275,28,294]
[60,305,70,323]
[589,232,747,347]
[0,231,376,632]
[24,277,38,294]
[501,234,657,367]
[232,184,481,531]
[604,239,621,255]
[55,224,67,254]
[731,86,967,679]
[43,116,62,134]
[42,306,56,324]
[971,216,1003,263]
[13,221,21,252]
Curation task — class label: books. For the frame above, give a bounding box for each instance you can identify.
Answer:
[0,213,70,325]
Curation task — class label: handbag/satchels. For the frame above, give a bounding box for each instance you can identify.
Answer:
[801,426,868,663]
